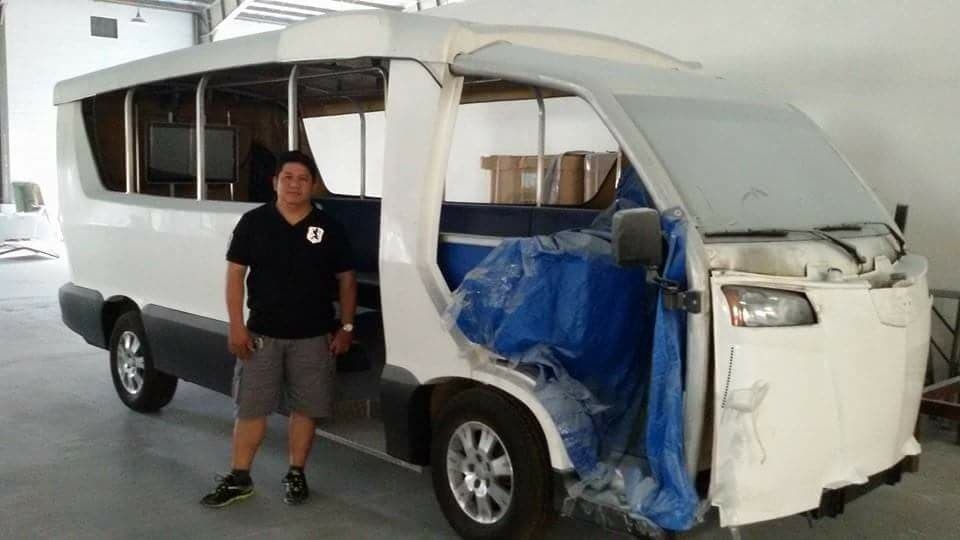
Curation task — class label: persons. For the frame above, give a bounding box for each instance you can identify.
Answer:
[197,150,356,508]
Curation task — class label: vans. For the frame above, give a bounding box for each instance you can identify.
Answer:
[53,9,936,540]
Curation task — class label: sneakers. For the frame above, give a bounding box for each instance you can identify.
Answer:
[199,471,255,509]
[279,471,309,505]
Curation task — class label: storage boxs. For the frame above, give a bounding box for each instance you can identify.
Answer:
[481,154,616,207]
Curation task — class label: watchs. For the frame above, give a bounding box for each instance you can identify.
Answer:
[341,321,354,333]
